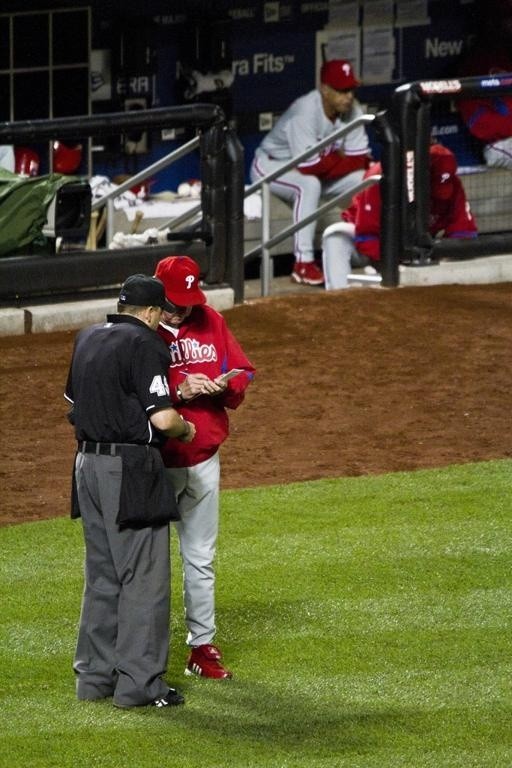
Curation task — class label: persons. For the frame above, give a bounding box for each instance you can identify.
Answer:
[339,143,477,263]
[150,254,259,680]
[61,272,200,710]
[247,59,385,286]
[453,39,512,175]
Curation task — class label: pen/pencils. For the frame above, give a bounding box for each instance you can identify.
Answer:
[178,370,190,376]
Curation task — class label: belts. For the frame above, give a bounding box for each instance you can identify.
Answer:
[77,441,127,456]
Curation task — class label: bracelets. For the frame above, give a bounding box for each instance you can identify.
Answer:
[175,384,185,402]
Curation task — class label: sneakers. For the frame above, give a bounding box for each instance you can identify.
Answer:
[148,688,185,707]
[184,645,231,679]
[292,262,324,285]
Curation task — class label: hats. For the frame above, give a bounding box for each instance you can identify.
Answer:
[429,144,456,199]
[119,256,206,312]
[321,60,359,91]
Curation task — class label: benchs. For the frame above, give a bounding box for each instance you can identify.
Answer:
[108,167,512,259]
